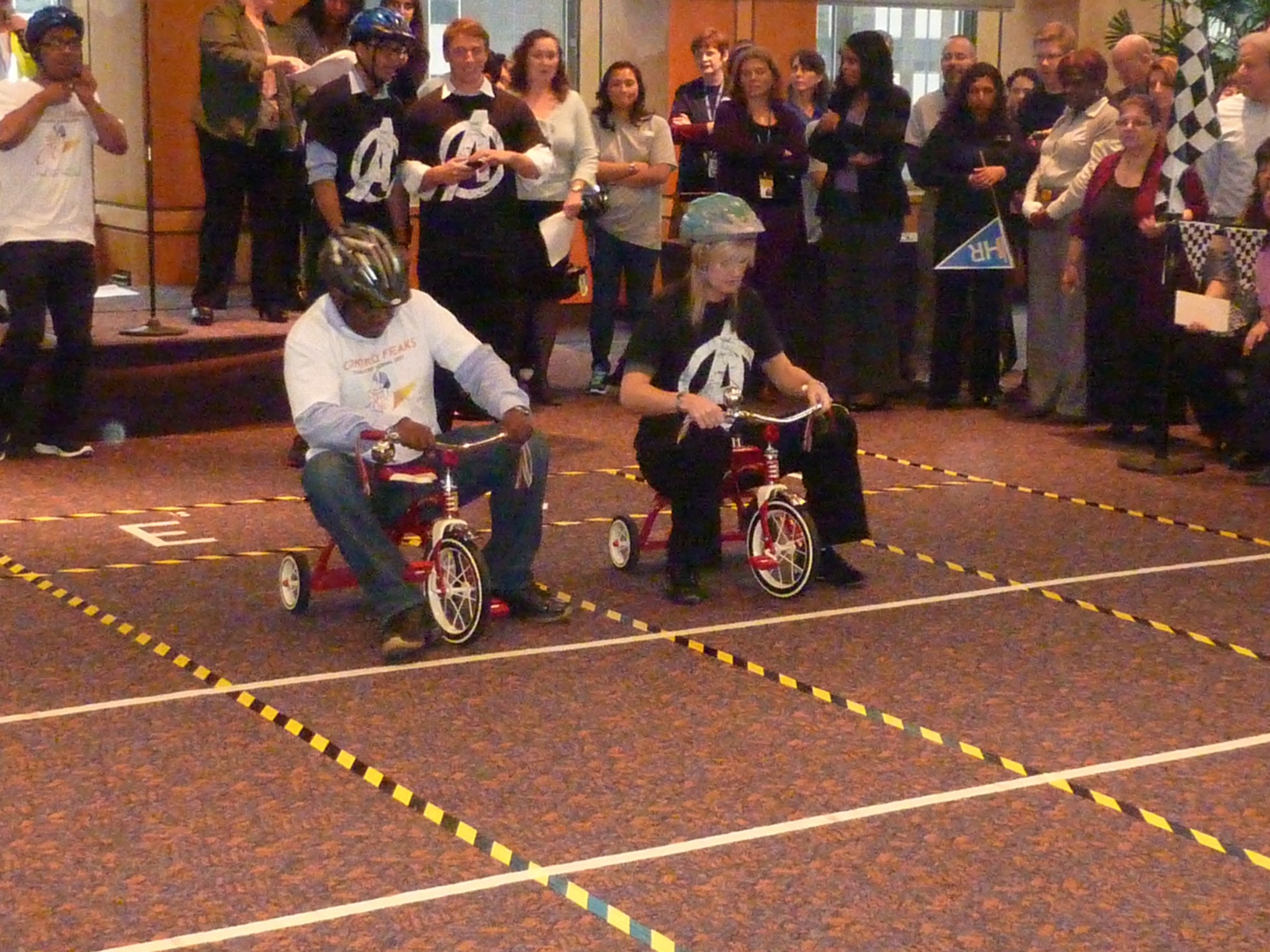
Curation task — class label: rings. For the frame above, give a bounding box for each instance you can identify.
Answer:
[984,182,988,184]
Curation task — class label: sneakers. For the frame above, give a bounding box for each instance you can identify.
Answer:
[588,370,610,395]
[33,432,96,458]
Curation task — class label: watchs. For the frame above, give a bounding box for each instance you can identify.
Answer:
[571,184,584,192]
[515,406,529,415]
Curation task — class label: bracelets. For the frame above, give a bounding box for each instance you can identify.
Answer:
[674,392,683,412]
[630,162,635,175]
[801,379,825,394]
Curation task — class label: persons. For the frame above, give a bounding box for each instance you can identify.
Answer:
[714,44,809,400]
[1060,95,1209,445]
[619,193,870,603]
[394,19,557,414]
[191,1,1270,486]
[0,4,129,462]
[507,28,600,404]
[1021,47,1123,424]
[805,31,911,407]
[914,60,1030,409]
[0,0,35,325]
[302,7,415,308]
[284,223,571,656]
[586,59,679,396]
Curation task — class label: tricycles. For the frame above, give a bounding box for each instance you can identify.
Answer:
[604,396,832,602]
[279,428,533,646]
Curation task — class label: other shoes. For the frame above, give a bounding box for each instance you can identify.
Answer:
[1230,453,1255,467]
[1246,470,1270,485]
[263,306,289,324]
[191,306,215,327]
[526,371,561,405]
[1048,412,1086,423]
[287,435,310,468]
[1130,425,1169,444]
[796,542,866,584]
[975,388,996,404]
[832,391,882,409]
[1022,407,1050,419]
[492,583,571,623]
[667,560,704,606]
[380,602,442,659]
[928,391,954,407]
[1097,422,1132,440]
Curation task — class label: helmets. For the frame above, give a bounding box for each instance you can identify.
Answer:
[317,223,411,309]
[346,7,416,47]
[24,6,85,49]
[677,192,766,245]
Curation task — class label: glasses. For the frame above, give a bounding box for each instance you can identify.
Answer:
[33,36,84,50]
[1115,119,1151,128]
[368,38,408,54]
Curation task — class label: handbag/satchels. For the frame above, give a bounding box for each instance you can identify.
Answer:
[577,190,608,220]
